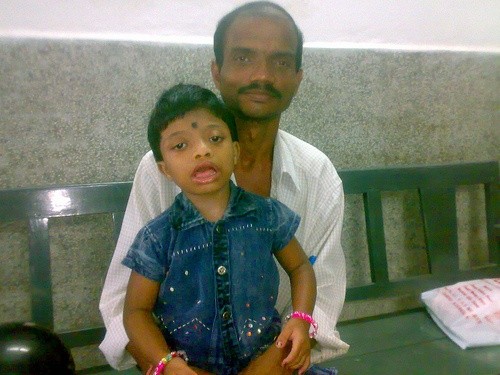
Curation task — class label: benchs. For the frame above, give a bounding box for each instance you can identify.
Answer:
[0,162,500,375]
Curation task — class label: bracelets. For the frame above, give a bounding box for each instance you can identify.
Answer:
[285,312,319,338]
[153,350,189,375]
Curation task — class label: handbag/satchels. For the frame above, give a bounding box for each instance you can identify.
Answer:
[420,276,500,350]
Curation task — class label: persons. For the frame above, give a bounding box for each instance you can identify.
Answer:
[120,83,339,375]
[98,0,351,374]
